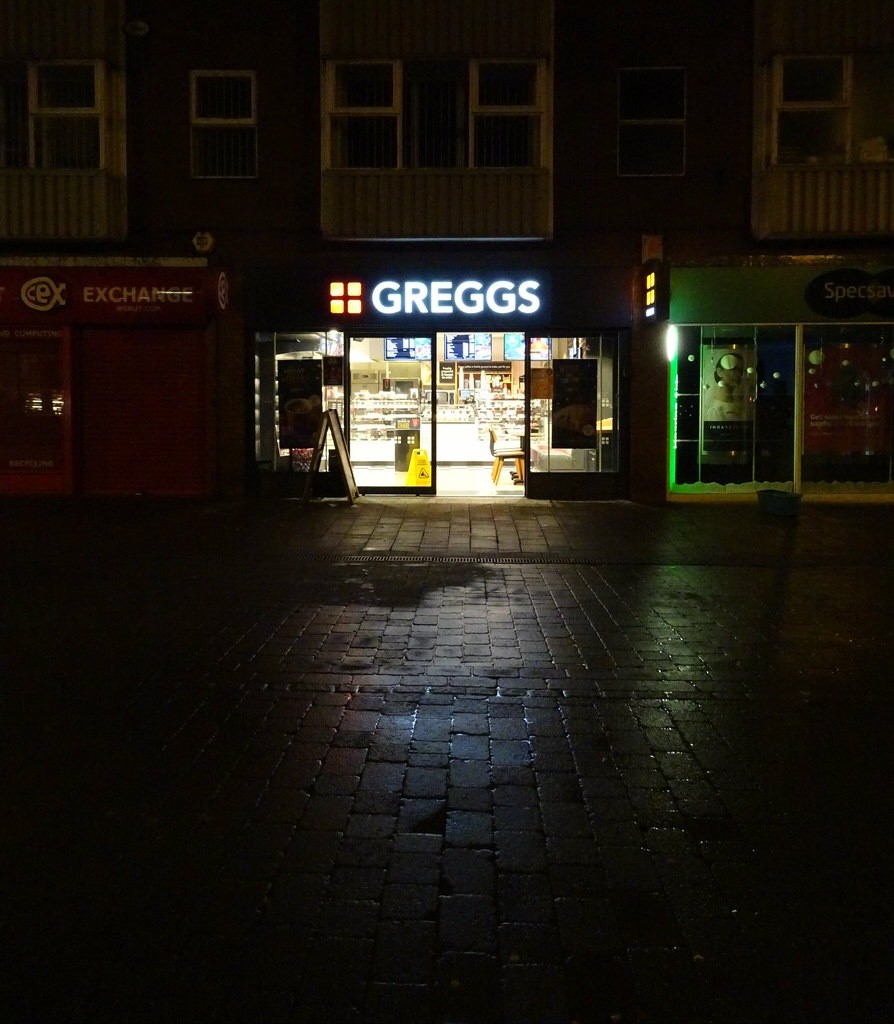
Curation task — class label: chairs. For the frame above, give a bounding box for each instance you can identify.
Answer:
[531,446,568,468]
[487,427,525,486]
[536,449,587,472]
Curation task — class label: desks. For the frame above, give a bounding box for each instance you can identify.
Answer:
[510,432,545,452]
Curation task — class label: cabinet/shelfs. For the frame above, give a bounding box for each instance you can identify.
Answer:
[350,398,421,466]
[475,398,546,443]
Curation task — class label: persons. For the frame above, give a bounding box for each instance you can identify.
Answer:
[708,352,747,421]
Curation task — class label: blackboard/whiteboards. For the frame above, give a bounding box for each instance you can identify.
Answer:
[300,408,358,499]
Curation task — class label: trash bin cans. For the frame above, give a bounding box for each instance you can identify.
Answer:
[394,429,420,472]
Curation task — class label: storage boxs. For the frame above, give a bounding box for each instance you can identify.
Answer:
[756,489,803,519]
